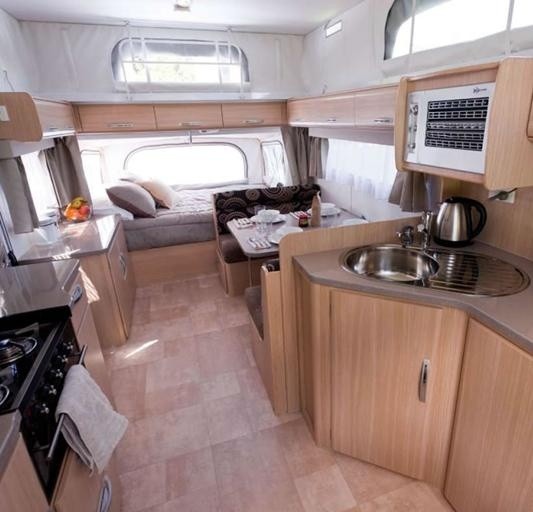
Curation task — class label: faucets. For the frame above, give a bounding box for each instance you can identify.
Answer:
[417,210,433,249]
[395,226,415,248]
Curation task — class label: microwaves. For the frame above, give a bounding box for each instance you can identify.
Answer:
[404,83,494,174]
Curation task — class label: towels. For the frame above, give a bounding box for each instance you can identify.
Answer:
[56,365,128,475]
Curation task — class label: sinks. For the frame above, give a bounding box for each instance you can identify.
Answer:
[346,248,439,282]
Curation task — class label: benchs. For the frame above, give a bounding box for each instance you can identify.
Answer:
[209,183,321,295]
[244,257,286,415]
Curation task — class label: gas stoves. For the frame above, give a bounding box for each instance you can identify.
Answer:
[0,304,78,412]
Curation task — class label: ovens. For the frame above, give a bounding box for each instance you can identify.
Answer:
[17,318,88,503]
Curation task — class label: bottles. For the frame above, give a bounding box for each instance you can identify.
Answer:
[311,191,322,225]
[299,215,308,227]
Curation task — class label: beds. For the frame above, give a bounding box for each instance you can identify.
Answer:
[121,182,269,287]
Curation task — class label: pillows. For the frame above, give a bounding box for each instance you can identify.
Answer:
[135,176,178,207]
[105,179,156,218]
[93,201,134,220]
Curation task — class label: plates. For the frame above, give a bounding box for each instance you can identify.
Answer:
[250,214,286,223]
[306,208,341,216]
[268,234,280,245]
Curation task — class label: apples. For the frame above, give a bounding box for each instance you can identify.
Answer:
[79,206,90,217]
[71,201,81,210]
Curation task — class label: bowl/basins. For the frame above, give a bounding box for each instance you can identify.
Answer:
[276,227,304,240]
[316,203,335,213]
[259,210,279,220]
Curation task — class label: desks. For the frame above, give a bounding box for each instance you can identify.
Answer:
[227,207,369,286]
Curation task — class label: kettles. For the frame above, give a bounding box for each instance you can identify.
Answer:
[433,197,487,248]
[432,251,480,290]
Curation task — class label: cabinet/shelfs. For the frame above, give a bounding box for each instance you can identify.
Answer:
[443,275,533,512]
[53,451,124,512]
[1,257,120,413]
[355,82,395,127]
[17,214,136,347]
[156,99,223,131]
[222,98,287,127]
[315,277,472,488]
[0,412,51,511]
[0,92,79,143]
[287,91,356,130]
[75,101,157,133]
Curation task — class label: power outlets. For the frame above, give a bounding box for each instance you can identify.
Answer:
[490,190,516,204]
